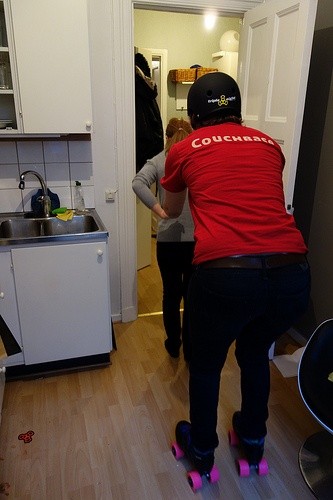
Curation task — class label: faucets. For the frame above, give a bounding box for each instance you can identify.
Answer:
[19,168,52,216]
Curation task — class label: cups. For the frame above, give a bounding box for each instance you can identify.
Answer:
[0,52,10,89]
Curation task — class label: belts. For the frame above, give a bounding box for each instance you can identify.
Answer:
[203,253,308,268]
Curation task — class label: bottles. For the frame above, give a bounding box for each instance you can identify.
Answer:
[74,180,87,215]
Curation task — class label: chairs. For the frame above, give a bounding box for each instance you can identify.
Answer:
[298,319,333,500]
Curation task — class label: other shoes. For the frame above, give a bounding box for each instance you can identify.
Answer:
[163,337,181,359]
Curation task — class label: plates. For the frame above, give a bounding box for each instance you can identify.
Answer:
[0,119,13,128]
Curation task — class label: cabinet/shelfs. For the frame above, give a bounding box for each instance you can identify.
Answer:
[0,237,112,382]
[0,0,92,141]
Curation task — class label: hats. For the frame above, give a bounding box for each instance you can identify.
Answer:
[186,71,242,121]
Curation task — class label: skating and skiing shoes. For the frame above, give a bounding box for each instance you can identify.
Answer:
[170,420,221,492]
[228,410,269,477]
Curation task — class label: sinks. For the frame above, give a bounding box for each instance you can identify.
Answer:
[0,208,109,245]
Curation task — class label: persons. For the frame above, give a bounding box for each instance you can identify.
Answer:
[131,117,194,358]
[162,72,308,490]
